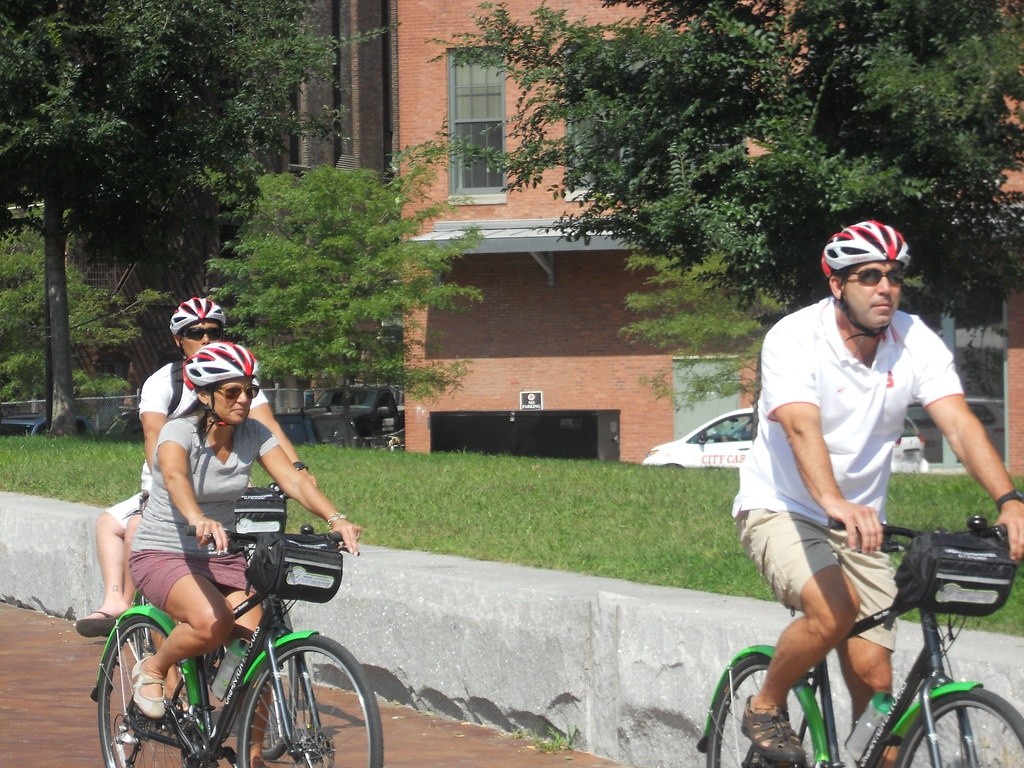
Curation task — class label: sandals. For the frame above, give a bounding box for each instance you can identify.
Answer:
[742,695,805,761]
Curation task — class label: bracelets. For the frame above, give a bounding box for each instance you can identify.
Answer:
[996,490,1024,512]
[328,513,348,531]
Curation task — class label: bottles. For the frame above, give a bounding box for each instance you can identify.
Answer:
[209,639,250,702]
[846,692,897,760]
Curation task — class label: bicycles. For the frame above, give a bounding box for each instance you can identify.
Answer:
[696,517,1024,768]
[88,523,386,768]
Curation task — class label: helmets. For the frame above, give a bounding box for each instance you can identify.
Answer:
[182,341,259,392]
[170,298,226,335]
[821,220,911,276]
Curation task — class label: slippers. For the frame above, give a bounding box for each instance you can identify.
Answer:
[76,611,116,636]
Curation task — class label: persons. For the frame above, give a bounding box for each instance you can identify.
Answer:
[75,297,317,638]
[730,220,1024,768]
[128,342,362,768]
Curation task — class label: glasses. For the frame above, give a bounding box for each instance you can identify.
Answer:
[212,384,261,399]
[843,269,904,286]
[180,327,223,341]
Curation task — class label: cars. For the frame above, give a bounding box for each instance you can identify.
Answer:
[304,384,404,441]
[901,398,1004,462]
[639,407,931,474]
[0,415,94,438]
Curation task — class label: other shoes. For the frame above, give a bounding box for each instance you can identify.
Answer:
[133,656,166,718]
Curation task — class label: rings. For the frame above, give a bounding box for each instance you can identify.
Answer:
[203,533,211,538]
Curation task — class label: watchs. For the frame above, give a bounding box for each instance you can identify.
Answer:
[294,461,309,470]
